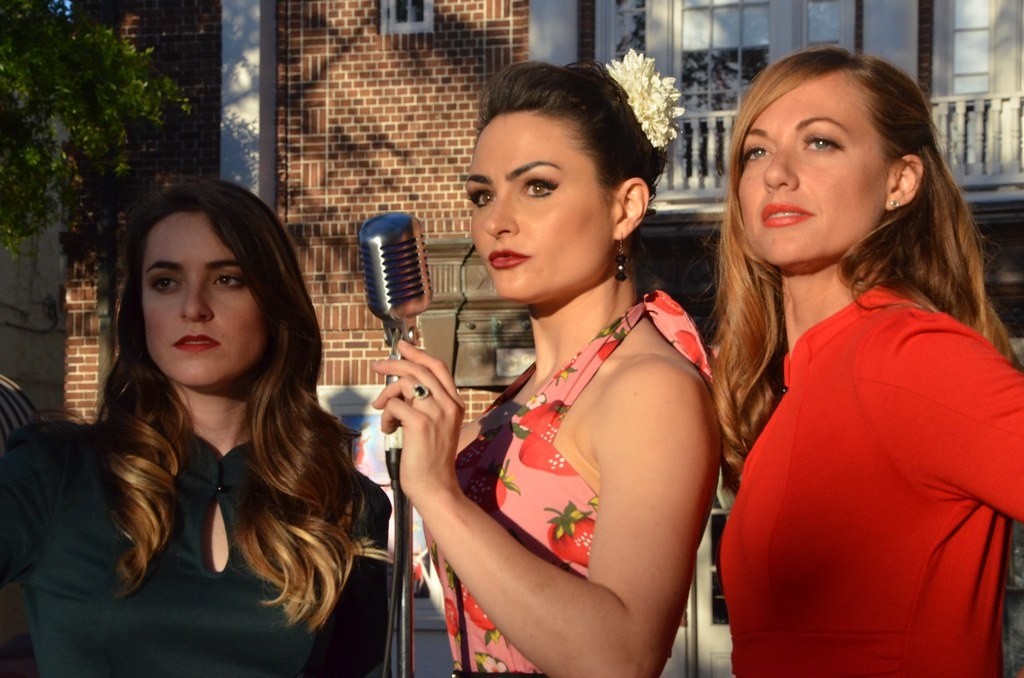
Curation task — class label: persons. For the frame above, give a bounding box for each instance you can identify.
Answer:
[708,44,1024,678]
[369,50,725,678]
[0,178,392,678]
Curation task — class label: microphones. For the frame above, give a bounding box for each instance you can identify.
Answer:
[358,211,434,490]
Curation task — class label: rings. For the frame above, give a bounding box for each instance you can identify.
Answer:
[410,383,429,406]
